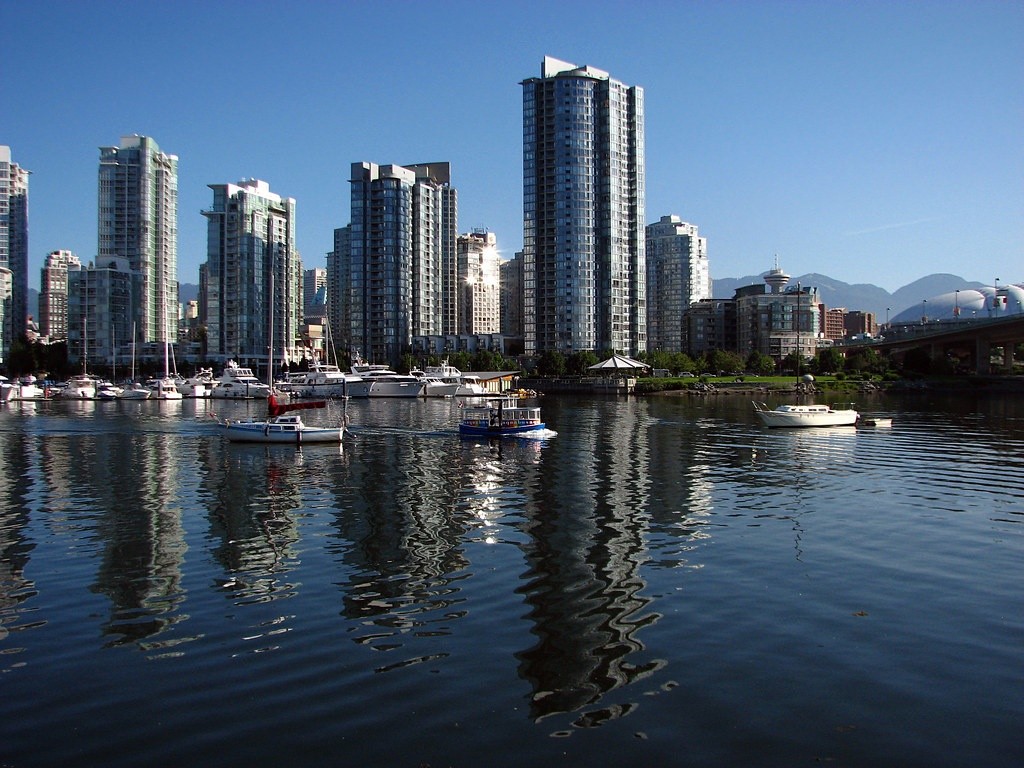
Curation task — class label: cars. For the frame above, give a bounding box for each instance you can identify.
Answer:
[679,372,694,377]
[700,372,716,377]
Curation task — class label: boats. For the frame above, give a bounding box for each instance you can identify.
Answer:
[409,353,476,397]
[96,384,118,398]
[209,359,270,399]
[273,307,376,398]
[346,350,425,398]
[21,374,57,399]
[459,400,545,438]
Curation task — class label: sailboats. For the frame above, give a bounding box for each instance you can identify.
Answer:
[150,306,206,399]
[65,317,96,400]
[210,273,349,444]
[751,281,860,428]
[104,321,151,401]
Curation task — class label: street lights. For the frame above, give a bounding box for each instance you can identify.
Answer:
[923,299,927,323]
[887,307,891,324]
[995,278,1000,318]
[956,289,960,320]
